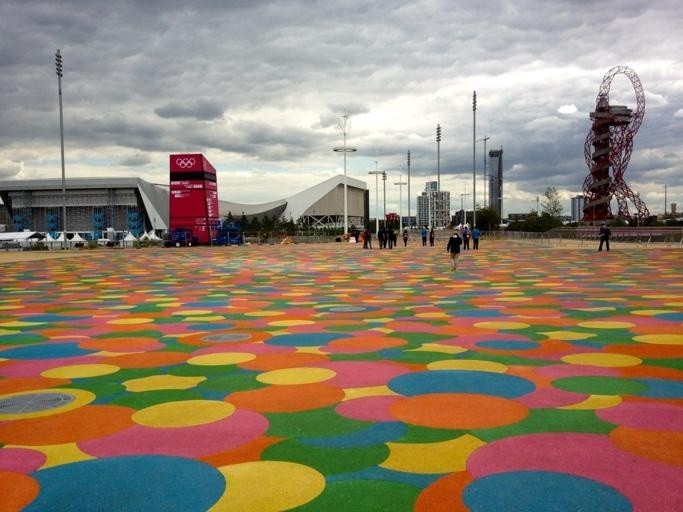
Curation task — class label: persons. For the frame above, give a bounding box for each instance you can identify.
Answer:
[470,225,480,249]
[597,224,610,252]
[402,230,408,246]
[377,229,384,248]
[446,233,462,272]
[392,227,399,247]
[363,231,367,248]
[429,227,434,246]
[382,227,389,248]
[421,228,427,247]
[362,225,372,249]
[460,225,464,236]
[462,227,470,250]
[386,225,393,249]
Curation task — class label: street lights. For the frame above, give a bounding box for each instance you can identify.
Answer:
[332,89,489,235]
[54,48,67,251]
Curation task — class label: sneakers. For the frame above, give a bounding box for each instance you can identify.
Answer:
[450,265,458,272]
